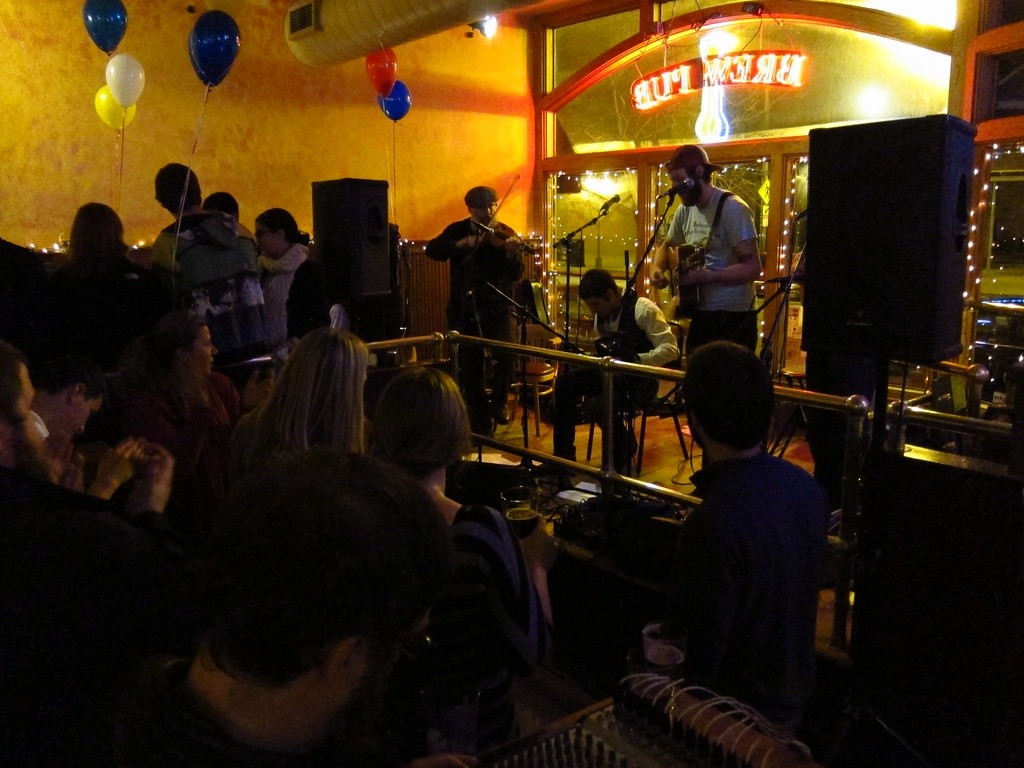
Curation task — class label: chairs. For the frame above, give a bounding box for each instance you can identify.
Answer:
[587,320,687,483]
[508,323,563,437]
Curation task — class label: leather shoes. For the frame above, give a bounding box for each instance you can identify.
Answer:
[494,403,511,425]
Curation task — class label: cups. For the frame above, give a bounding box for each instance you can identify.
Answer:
[643,624,688,680]
[501,486,542,539]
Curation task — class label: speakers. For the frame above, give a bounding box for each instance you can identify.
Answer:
[312,177,391,296]
[800,114,978,365]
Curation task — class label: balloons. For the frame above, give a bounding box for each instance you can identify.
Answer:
[187,10,241,87]
[83,0,128,53]
[378,80,411,123]
[94,86,137,130]
[366,46,399,97]
[105,52,145,108]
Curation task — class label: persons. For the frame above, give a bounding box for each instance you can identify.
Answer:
[647,342,830,738]
[805,352,890,490]
[0,318,242,768]
[25,163,350,370]
[231,330,369,466]
[426,186,526,424]
[374,365,558,757]
[650,145,762,354]
[122,445,479,768]
[538,269,680,473]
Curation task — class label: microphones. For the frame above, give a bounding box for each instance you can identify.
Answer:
[601,194,620,210]
[658,178,695,199]
[766,274,802,283]
[472,220,495,236]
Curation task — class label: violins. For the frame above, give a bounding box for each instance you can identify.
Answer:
[475,216,537,257]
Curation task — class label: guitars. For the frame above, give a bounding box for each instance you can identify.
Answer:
[668,243,707,307]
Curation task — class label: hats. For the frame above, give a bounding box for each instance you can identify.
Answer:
[665,144,721,172]
[464,186,498,209]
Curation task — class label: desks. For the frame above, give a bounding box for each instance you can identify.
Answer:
[498,473,692,591]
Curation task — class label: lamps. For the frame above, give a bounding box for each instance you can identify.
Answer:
[468,17,498,38]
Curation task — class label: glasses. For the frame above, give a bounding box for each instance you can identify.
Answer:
[254,228,279,239]
